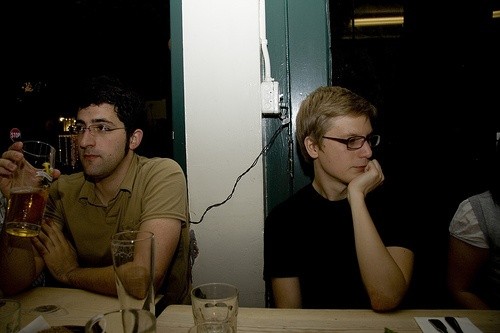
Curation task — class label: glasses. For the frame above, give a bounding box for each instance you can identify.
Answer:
[72,123,127,137]
[322,135,380,150]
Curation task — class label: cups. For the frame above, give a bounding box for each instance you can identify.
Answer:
[4,142,55,238]
[110,230,156,318]
[188,321,233,333]
[190,283,238,333]
[84,308,157,333]
[0,300,21,333]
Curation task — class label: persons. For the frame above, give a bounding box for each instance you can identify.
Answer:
[0,86,190,319]
[262,85,415,311]
[446,122,500,310]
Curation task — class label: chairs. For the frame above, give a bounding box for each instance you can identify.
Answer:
[156,228,198,313]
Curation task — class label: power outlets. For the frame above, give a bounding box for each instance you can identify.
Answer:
[261,81,282,115]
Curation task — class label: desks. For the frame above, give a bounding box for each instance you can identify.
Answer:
[156,301,500,333]
[0,284,155,333]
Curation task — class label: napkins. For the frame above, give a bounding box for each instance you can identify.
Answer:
[414,315,483,333]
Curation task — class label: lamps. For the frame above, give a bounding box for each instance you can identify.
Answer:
[346,15,406,31]
[488,8,500,20]
[352,4,405,15]
